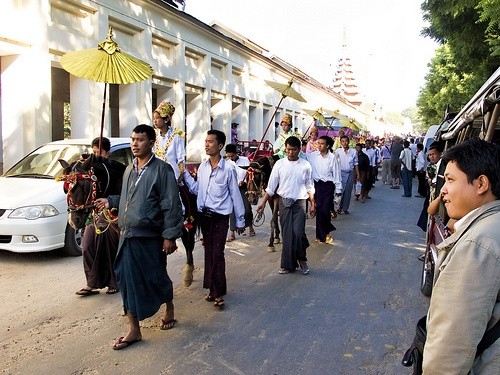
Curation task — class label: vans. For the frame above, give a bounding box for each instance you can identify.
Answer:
[423,124,441,152]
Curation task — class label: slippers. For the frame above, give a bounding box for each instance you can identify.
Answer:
[205,293,215,301]
[113,337,142,350]
[76,288,100,295]
[160,318,177,329]
[214,299,224,306]
[106,288,118,294]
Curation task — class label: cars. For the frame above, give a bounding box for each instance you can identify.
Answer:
[0,138,132,257]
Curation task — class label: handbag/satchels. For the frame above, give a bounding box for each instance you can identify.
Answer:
[402,315,482,375]
[355,180,362,195]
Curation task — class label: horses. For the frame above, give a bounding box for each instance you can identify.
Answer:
[236,151,281,253]
[56,152,202,289]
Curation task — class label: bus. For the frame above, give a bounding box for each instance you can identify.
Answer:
[315,114,353,139]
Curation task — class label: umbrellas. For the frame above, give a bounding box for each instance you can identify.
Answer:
[260,75,307,142]
[60,27,154,155]
[301,107,363,141]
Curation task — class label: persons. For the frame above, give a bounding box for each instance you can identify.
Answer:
[225,145,255,242]
[422,138,500,375]
[93,124,185,350]
[256,136,315,274]
[426,142,449,202]
[152,100,185,181]
[181,129,245,308]
[272,113,302,162]
[369,135,417,198]
[275,122,279,140]
[252,139,257,144]
[305,127,370,244]
[263,140,272,150]
[76,137,125,295]
[427,189,450,226]
[415,143,428,198]
[295,127,298,132]
[231,123,239,143]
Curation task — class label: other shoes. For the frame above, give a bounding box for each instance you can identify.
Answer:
[302,264,310,274]
[420,196,425,198]
[354,198,359,200]
[344,210,349,214]
[250,228,255,236]
[418,254,426,261]
[279,268,295,274]
[336,210,342,215]
[226,237,235,242]
[415,195,421,197]
[366,196,371,199]
[371,179,402,189]
[361,199,365,203]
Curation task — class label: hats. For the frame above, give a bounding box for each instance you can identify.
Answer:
[339,128,344,132]
[155,100,175,120]
[310,126,319,135]
[281,113,292,125]
